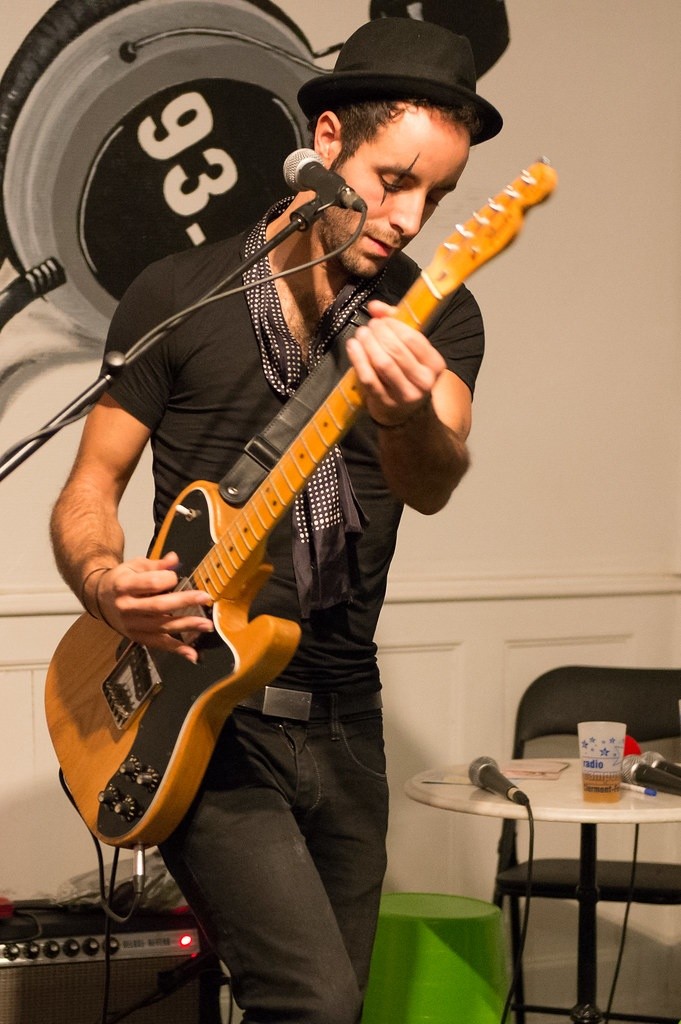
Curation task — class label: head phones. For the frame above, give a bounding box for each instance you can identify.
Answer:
[0,0,345,347]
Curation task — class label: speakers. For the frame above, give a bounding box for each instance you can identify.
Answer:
[0,899,221,1024]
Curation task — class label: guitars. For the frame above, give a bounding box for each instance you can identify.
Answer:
[41,154,559,854]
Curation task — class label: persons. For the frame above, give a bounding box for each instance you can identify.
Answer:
[48,16,502,1024]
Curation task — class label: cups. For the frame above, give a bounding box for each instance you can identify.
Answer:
[578,722,627,803]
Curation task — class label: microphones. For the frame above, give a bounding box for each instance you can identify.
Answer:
[468,757,532,805]
[284,149,370,213]
[622,751,681,797]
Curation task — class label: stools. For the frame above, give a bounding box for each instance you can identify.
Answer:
[360,892,510,1024]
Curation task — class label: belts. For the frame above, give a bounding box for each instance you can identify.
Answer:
[229,683,387,723]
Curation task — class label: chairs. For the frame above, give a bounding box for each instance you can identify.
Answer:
[493,663,681,1024]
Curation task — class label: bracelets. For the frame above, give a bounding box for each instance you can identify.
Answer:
[81,567,124,637]
[369,414,407,432]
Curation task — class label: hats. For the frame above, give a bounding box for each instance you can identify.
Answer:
[294,18,504,147]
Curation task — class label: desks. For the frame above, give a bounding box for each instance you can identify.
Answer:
[404,756,681,1024]
